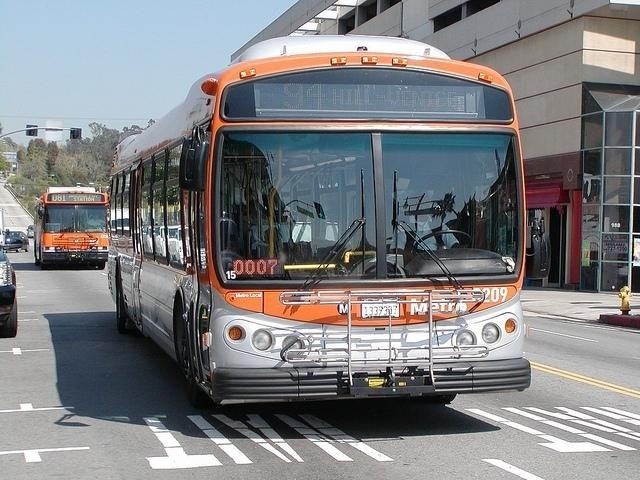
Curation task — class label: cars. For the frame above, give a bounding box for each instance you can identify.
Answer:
[109,216,207,272]
[2,229,31,253]
[26,224,34,238]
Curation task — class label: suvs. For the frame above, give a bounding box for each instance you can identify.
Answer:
[0,248,21,340]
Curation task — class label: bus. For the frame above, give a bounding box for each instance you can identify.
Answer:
[32,182,110,272]
[103,27,545,420]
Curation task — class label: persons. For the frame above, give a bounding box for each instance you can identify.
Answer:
[391,192,460,266]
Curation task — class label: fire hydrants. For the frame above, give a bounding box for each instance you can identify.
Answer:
[618,284,634,317]
